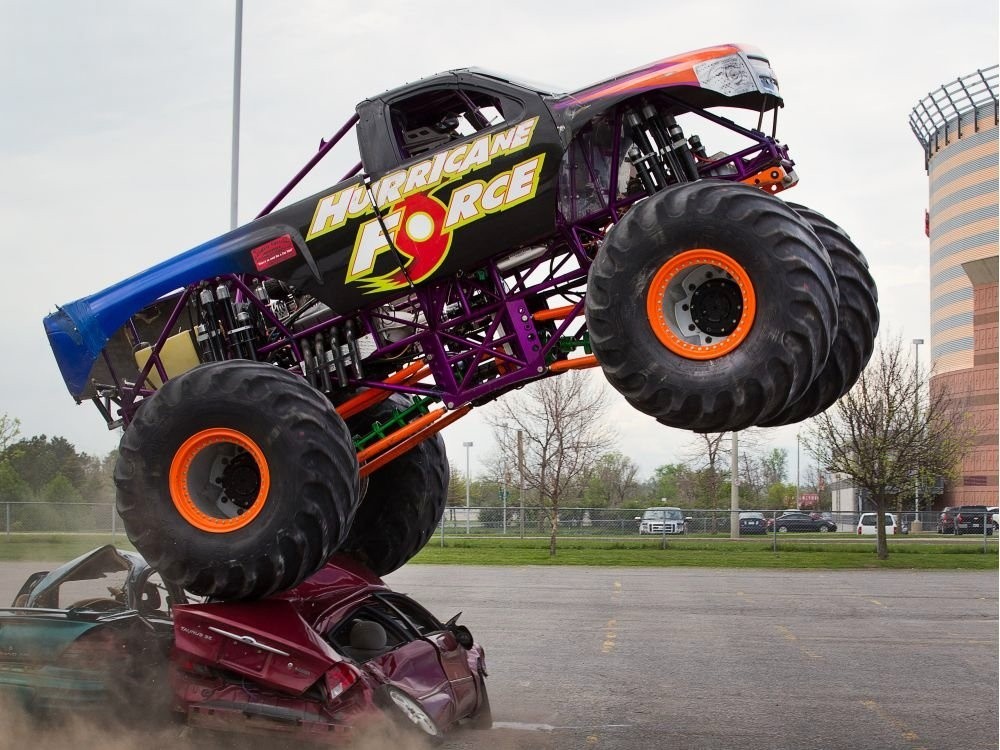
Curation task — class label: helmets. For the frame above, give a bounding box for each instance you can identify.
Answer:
[432,111,462,133]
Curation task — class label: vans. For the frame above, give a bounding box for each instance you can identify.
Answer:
[857,513,897,535]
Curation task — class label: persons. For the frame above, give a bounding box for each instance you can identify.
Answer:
[397,103,465,161]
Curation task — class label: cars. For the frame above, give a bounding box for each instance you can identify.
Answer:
[768,512,838,533]
[738,512,767,534]
[821,511,831,520]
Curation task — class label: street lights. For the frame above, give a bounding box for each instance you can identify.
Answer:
[463,442,473,534]
[912,339,923,524]
[501,423,508,534]
[797,433,800,510]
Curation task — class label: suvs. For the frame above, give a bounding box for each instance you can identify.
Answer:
[986,506,999,530]
[635,506,692,535]
[42,36,884,606]
[937,505,993,536]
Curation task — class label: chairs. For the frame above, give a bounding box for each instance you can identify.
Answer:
[343,621,393,661]
[142,581,166,617]
